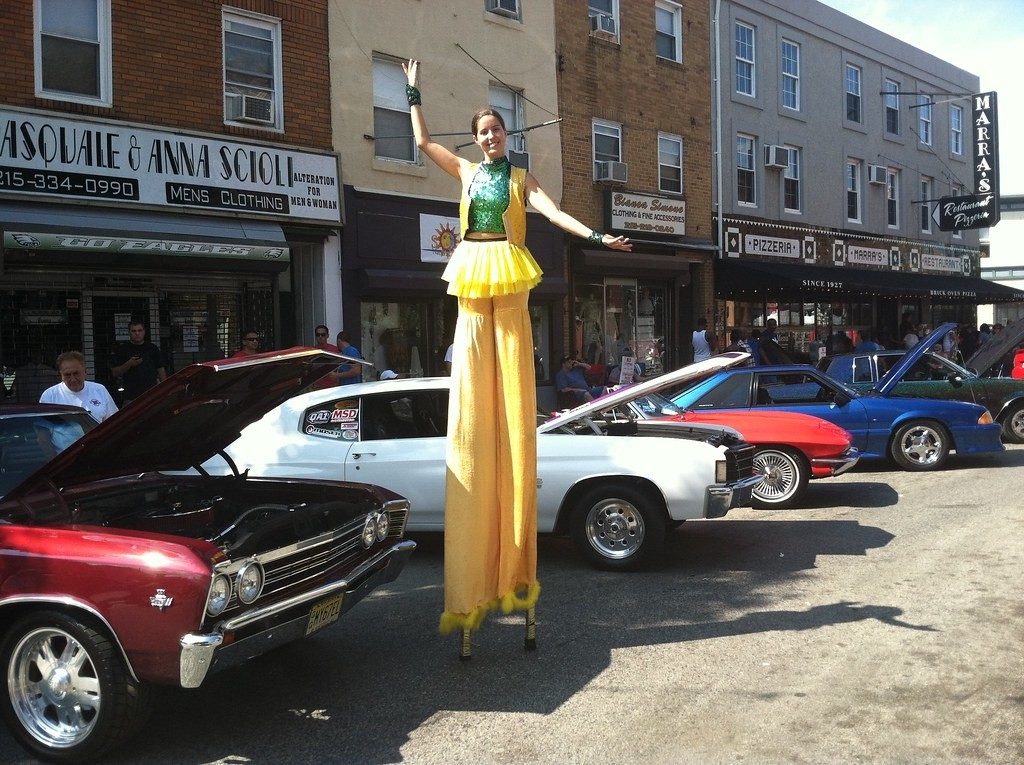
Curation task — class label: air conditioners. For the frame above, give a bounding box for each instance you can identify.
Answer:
[595,160,628,184]
[488,0,518,18]
[504,149,529,173]
[764,144,789,172]
[232,94,274,125]
[867,164,888,185]
[591,14,616,37]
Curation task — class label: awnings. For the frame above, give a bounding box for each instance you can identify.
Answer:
[713,258,1024,304]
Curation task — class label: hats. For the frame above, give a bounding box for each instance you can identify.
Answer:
[560,354,575,365]
[1007,319,1015,325]
[380,369,399,381]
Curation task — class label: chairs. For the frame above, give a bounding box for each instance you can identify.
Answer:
[368,397,448,440]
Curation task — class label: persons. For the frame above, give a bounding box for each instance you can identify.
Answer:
[401,58,633,624]
[445,344,453,376]
[579,291,633,364]
[34,351,119,463]
[852,313,1013,376]
[555,348,647,405]
[690,318,715,362]
[313,325,341,388]
[330,331,361,385]
[111,319,167,407]
[232,331,259,357]
[722,319,777,384]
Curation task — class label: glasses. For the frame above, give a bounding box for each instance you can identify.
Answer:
[315,333,327,337]
[935,350,939,351]
[994,328,1000,329]
[244,338,261,341]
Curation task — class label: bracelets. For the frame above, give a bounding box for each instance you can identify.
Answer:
[405,84,422,106]
[588,230,604,245]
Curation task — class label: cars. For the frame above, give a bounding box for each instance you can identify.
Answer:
[536,383,859,507]
[165,350,766,574]
[633,323,1007,471]
[0,345,416,765]
[812,315,1024,443]
